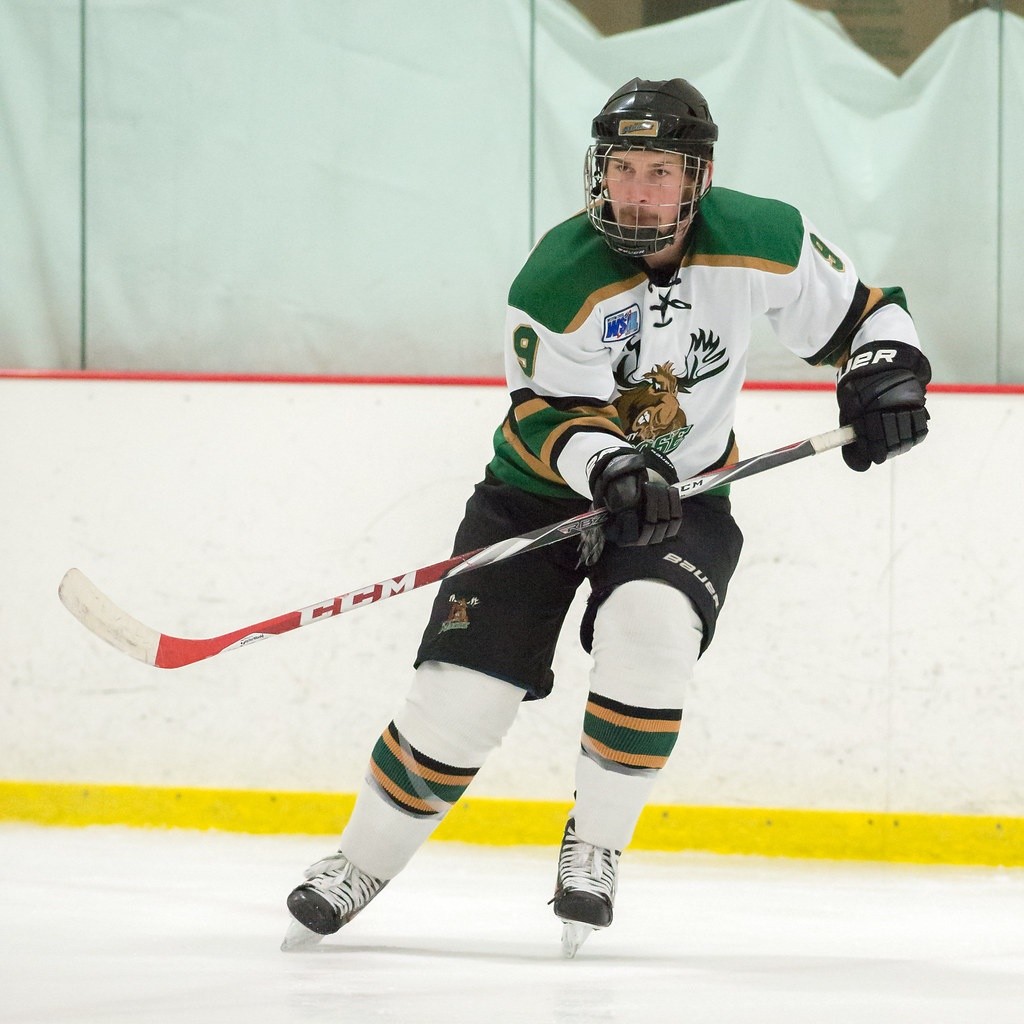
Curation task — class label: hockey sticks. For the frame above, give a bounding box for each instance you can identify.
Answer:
[55,423,856,670]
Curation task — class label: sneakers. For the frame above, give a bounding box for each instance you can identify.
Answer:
[279,850,389,951]
[547,817,623,959]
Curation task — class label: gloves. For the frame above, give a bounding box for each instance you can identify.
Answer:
[836,340,932,472]
[585,446,684,548]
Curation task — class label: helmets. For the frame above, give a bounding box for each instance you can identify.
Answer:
[583,77,719,259]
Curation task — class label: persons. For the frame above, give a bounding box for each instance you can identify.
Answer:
[288,75,931,935]
